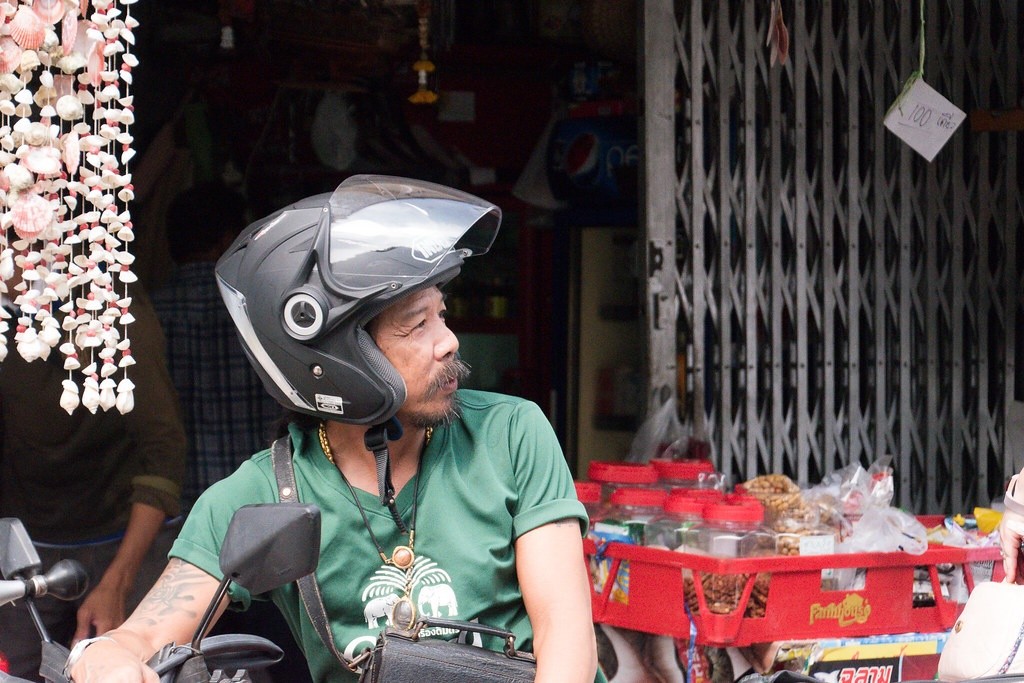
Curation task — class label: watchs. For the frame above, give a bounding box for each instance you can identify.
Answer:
[63,637,116,683]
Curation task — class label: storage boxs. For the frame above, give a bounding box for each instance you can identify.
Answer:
[582,514,1006,648]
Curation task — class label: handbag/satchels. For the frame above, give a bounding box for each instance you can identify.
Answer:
[359,616,536,683]
[937,473,1024,682]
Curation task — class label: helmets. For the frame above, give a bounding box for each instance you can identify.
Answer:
[214,173,464,426]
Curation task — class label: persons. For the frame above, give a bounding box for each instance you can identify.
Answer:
[0,241,187,683]
[62,173,598,683]
